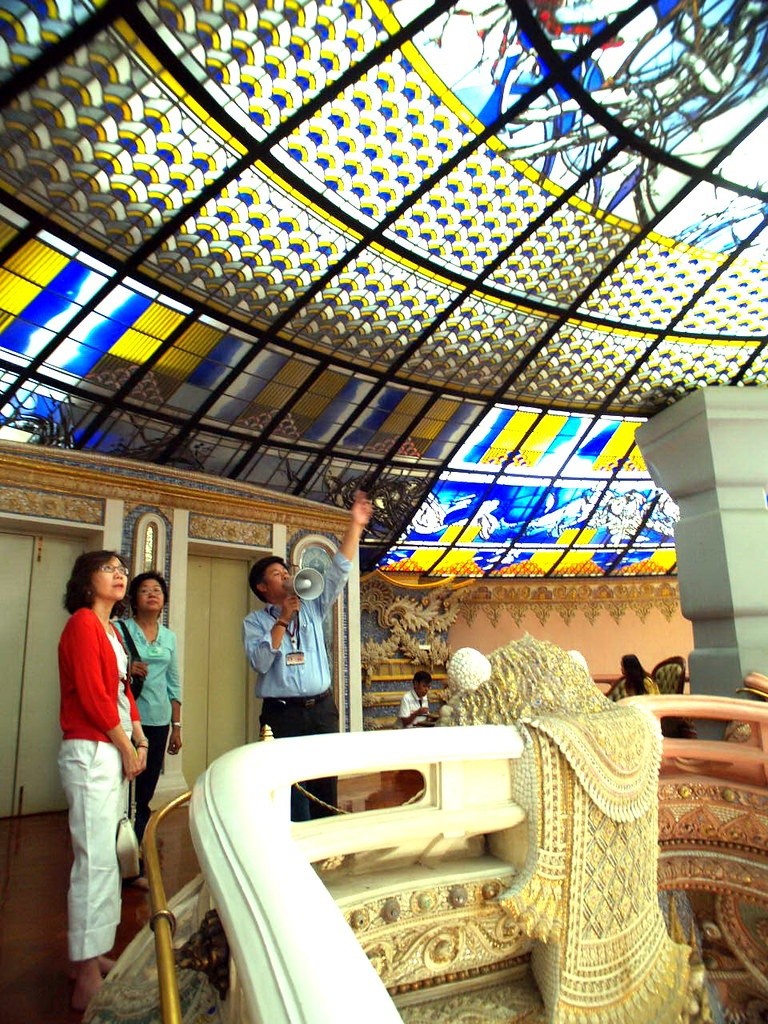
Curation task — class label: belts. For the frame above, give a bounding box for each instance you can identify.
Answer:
[264,689,330,709]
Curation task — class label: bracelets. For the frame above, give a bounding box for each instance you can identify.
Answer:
[137,745,148,749]
[172,723,182,727]
[275,619,288,628]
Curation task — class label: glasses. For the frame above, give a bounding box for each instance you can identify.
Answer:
[99,563,129,575]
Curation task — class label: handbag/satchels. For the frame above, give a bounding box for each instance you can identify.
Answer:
[118,620,145,699]
[115,759,140,878]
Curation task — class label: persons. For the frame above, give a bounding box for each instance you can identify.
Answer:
[56,550,149,1011]
[241,494,375,823]
[621,654,660,698]
[113,571,184,889]
[398,671,432,729]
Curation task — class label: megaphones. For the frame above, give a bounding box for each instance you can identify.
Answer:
[282,568,324,620]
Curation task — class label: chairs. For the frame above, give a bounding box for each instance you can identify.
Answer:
[603,657,687,737]
